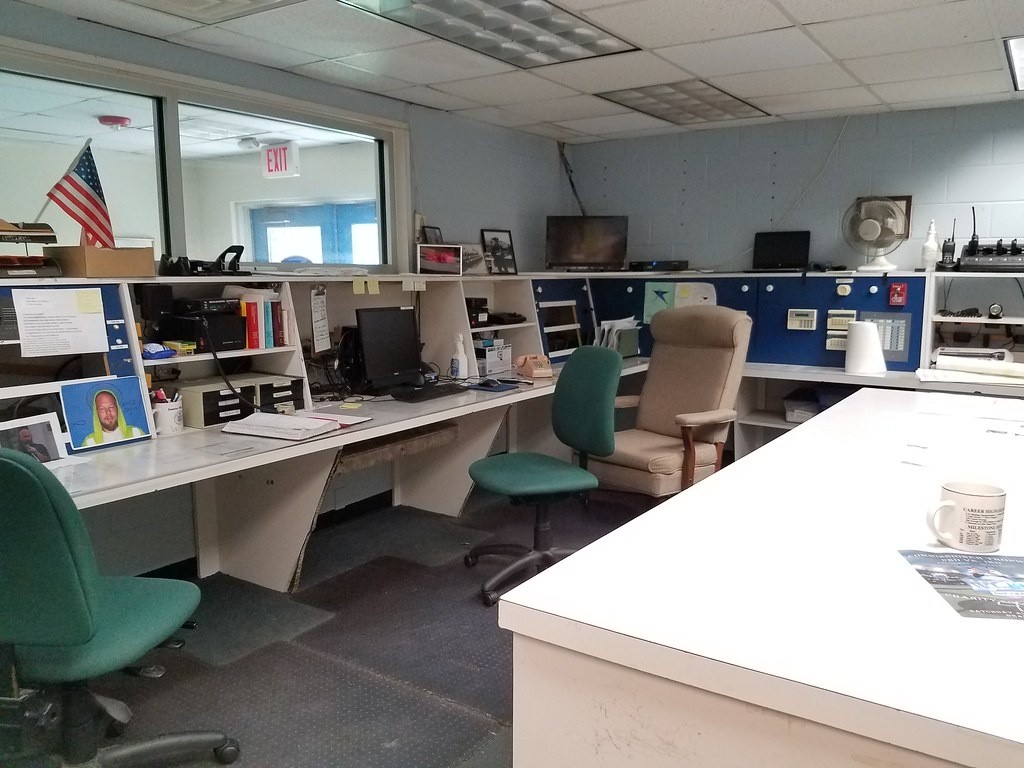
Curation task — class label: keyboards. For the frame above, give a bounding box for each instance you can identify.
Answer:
[391,384,470,402]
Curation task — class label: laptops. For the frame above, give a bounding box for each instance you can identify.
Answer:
[544,216,628,270]
[743,231,810,272]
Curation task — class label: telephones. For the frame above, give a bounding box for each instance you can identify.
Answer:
[514,354,552,378]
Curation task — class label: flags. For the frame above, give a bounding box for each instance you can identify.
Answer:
[46,145,115,249]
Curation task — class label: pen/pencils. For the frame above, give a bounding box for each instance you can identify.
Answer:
[148,386,182,402]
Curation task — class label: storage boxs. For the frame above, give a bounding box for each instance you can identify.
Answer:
[262,399,305,412]
[204,396,256,416]
[157,316,247,353]
[783,398,819,422]
[203,385,255,404]
[475,344,512,375]
[261,390,304,406]
[260,379,303,397]
[600,328,639,357]
[204,410,256,427]
[151,372,305,429]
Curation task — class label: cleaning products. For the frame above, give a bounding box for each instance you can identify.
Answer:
[448,333,469,378]
[920,218,942,270]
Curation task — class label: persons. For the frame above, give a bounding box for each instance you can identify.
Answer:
[491,237,509,274]
[81,389,147,448]
[16,426,51,464]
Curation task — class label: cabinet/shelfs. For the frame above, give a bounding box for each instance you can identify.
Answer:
[925,272,1024,369]
[419,279,545,377]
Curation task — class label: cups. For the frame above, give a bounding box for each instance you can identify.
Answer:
[151,401,183,434]
[927,481,1007,553]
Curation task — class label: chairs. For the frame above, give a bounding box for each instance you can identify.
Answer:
[581,304,755,512]
[0,447,242,768]
[463,344,624,606]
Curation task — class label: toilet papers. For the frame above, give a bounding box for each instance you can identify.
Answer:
[844,320,887,375]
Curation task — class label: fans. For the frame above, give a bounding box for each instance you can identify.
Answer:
[842,195,908,273]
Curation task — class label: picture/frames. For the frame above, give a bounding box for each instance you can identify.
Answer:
[481,229,517,275]
[0,411,72,471]
[416,244,462,277]
[445,242,489,275]
[422,225,444,244]
[854,194,912,240]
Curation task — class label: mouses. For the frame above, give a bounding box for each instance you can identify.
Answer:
[478,378,501,388]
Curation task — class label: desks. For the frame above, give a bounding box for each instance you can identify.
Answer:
[45,361,1024,768]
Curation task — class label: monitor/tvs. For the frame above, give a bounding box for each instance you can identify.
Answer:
[356,305,421,395]
